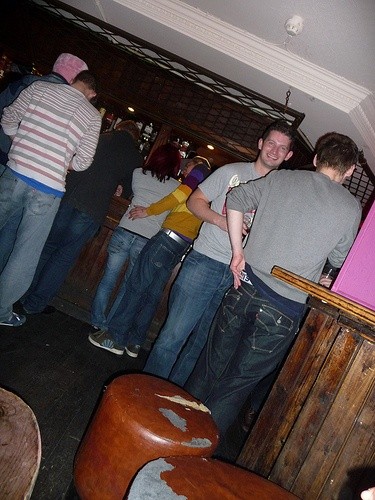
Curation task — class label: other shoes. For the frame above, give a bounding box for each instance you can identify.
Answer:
[90,322,102,333]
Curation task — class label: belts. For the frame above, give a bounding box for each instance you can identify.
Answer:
[159,223,189,249]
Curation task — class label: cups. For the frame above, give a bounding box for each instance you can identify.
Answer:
[320,265,335,287]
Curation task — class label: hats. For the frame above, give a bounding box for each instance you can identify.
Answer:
[53,53,89,84]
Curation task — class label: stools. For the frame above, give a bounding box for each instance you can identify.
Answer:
[70,374,219,500]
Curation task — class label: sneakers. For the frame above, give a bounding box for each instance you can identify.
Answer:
[90,329,141,357]
[0,311,27,328]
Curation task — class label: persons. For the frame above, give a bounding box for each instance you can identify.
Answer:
[16,119,142,317]
[183,131,363,459]
[90,140,179,330]
[1,53,90,172]
[89,159,211,360]
[0,70,102,326]
[141,118,293,388]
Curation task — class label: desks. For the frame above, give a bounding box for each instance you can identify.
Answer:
[125,454,304,500]
